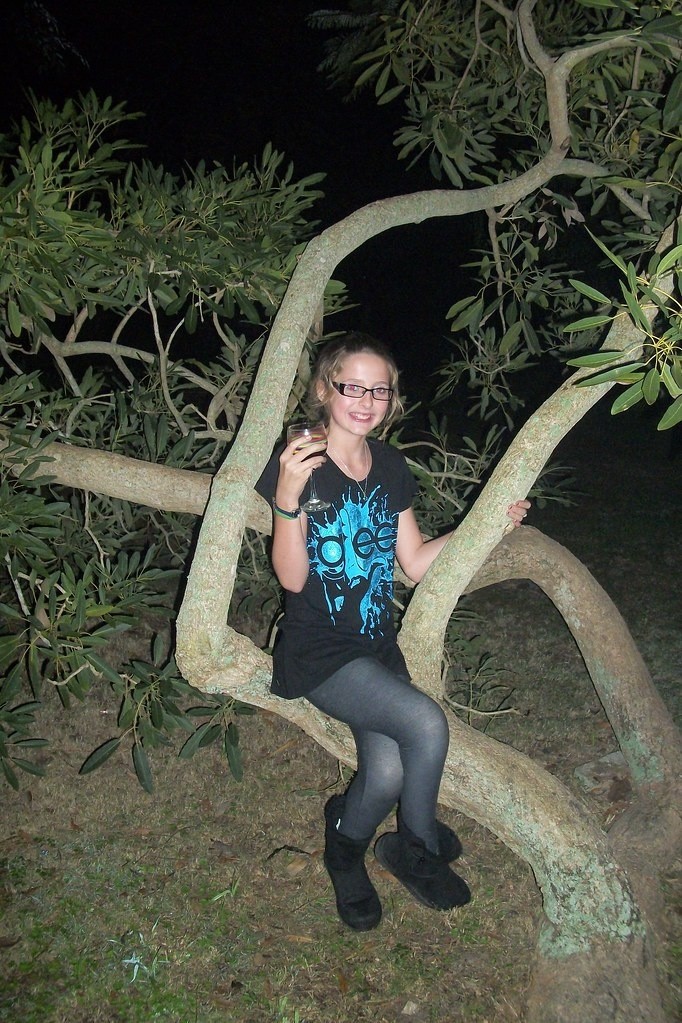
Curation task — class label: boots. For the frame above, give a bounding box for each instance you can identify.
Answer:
[323,795,382,931]
[374,807,473,912]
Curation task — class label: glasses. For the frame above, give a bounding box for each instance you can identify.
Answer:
[329,379,395,401]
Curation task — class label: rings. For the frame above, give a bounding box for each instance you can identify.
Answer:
[523,512,527,517]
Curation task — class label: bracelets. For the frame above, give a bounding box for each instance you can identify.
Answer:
[273,498,301,519]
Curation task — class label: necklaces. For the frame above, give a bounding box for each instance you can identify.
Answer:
[331,443,369,500]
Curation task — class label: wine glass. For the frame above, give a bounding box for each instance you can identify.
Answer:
[286,422,331,512]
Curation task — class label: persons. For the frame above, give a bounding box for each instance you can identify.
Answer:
[271,333,531,932]
